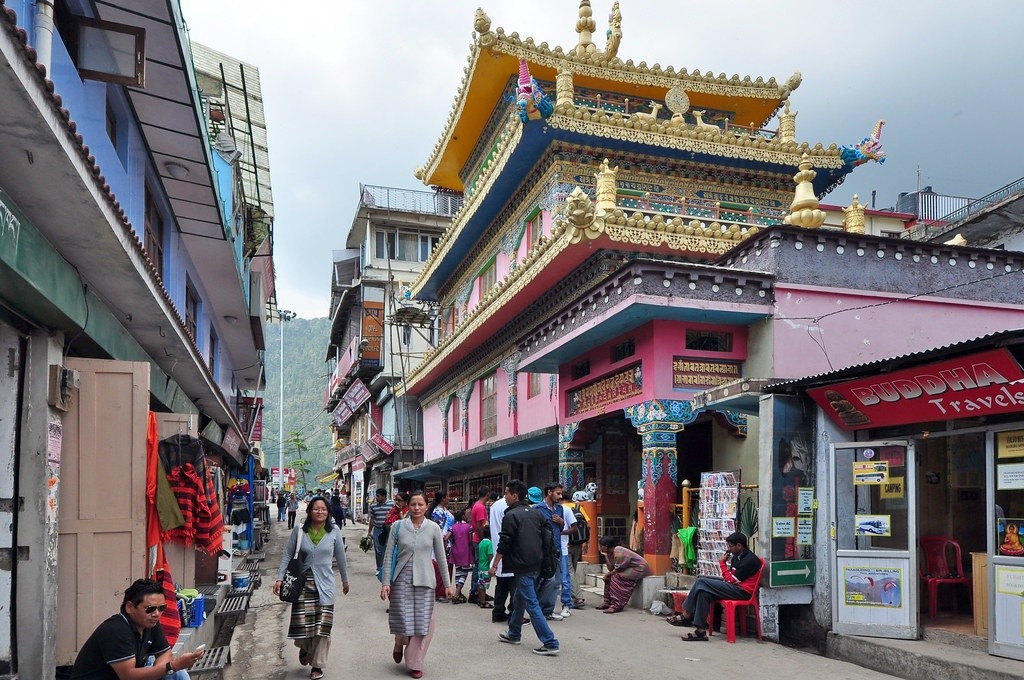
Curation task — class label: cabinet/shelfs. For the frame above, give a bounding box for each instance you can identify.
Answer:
[969,551,988,635]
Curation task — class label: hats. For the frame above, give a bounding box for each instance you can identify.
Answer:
[528,487,542,503]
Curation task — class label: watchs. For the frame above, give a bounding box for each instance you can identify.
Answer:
[166,662,175,675]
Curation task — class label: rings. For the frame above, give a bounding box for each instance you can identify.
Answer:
[194,657,198,662]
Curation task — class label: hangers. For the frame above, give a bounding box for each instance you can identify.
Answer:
[177,428,184,440]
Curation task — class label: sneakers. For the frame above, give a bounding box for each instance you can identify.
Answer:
[546,613,563,620]
[498,632,520,644]
[561,606,570,618]
[533,645,559,654]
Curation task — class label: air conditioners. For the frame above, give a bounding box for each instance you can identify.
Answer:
[397,462,412,469]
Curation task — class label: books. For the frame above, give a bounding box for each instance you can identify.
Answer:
[697,472,738,577]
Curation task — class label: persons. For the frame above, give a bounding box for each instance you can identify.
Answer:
[427,482,591,626]
[304,488,355,530]
[285,493,299,529]
[489,478,561,657]
[274,497,350,679]
[667,533,762,641]
[367,487,408,614]
[266,486,285,503]
[778,422,812,487]
[277,493,287,521]
[380,491,455,679]
[630,509,638,550]
[69,579,204,680]
[595,537,649,613]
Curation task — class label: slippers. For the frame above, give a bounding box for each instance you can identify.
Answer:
[572,601,584,608]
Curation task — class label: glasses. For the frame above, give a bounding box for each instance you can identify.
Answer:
[137,602,167,614]
[394,498,405,502]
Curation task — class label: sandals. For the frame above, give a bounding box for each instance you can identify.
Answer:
[666,614,693,627]
[682,630,709,641]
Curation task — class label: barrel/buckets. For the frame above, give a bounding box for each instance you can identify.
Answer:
[240,540,249,549]
[231,571,250,591]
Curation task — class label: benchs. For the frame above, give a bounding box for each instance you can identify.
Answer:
[657,590,690,613]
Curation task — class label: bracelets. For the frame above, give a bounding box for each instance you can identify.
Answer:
[492,565,498,568]
[399,512,402,514]
[275,581,282,582]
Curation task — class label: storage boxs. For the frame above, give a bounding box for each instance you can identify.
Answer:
[176,594,206,627]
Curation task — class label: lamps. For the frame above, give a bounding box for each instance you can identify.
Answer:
[224,315,238,325]
[245,378,255,384]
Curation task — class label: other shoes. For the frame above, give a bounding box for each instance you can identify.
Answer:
[411,670,422,677]
[393,643,403,663]
[376,571,380,576]
[523,618,530,625]
[310,668,323,679]
[502,614,508,621]
[436,589,495,608]
[595,601,608,609]
[299,649,309,665]
[603,608,616,612]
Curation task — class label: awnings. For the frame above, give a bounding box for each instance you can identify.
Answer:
[762,327,1024,390]
[265,297,280,324]
[191,38,274,227]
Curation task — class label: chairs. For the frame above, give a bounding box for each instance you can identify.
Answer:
[920,535,972,621]
[708,557,766,642]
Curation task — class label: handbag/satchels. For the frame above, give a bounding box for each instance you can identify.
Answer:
[279,523,305,603]
[376,520,401,585]
[378,524,388,546]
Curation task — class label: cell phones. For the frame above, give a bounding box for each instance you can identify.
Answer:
[193,643,206,653]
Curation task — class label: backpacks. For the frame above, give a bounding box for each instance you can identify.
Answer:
[568,503,589,544]
[535,509,560,579]
